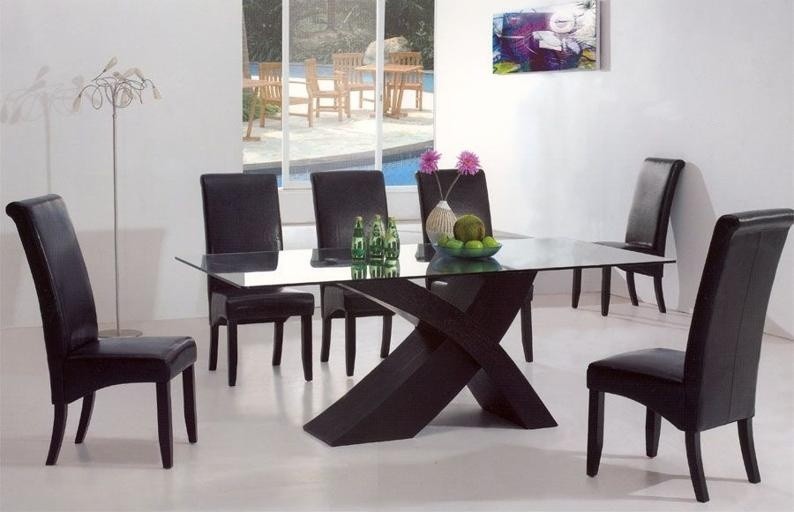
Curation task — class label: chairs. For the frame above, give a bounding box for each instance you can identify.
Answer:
[310,169,397,377]
[587,208,794,503]
[200,173,314,387]
[6,194,198,469]
[416,168,534,362]
[571,157,684,316]
[258,52,424,128]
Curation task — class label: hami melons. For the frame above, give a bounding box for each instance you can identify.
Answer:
[453,214,485,243]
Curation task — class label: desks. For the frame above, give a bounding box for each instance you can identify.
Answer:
[172,237,676,448]
[242,79,268,142]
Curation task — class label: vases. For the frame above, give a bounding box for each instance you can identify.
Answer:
[426,200,455,252]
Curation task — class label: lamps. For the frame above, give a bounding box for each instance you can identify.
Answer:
[73,56,162,342]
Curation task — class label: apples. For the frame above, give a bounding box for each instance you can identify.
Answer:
[437,237,450,246]
[464,241,482,257]
[482,236,498,254]
[446,240,464,256]
[433,257,496,273]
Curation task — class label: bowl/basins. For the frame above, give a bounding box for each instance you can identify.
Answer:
[428,256,502,277]
[433,243,502,259]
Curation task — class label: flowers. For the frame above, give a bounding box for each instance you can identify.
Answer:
[419,148,482,202]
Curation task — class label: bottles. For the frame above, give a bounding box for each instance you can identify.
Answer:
[351,215,400,263]
[350,260,399,282]
